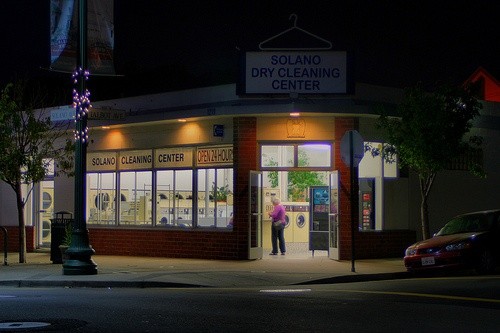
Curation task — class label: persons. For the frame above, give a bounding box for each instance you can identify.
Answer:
[157,216,170,227]
[268,197,287,255]
[225,217,233,231]
[177,217,187,229]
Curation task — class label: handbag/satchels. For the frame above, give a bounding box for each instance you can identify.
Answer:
[274,220,284,231]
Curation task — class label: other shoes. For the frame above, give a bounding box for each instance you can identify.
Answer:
[270,252,277,255]
[281,252,285,254]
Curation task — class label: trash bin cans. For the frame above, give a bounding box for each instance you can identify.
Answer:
[49,211,74,264]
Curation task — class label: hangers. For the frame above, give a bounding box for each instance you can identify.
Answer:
[258,13,333,50]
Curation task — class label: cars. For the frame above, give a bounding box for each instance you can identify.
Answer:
[403,208,500,277]
